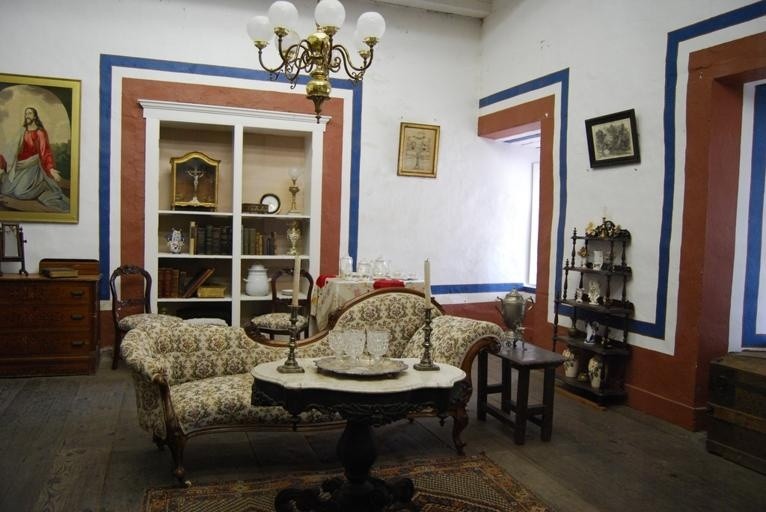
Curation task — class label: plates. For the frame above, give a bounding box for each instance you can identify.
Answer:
[260,194,281,214]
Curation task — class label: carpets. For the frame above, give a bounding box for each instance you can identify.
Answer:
[140,449,552,512]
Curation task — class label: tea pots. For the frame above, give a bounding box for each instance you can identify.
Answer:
[368,255,393,277]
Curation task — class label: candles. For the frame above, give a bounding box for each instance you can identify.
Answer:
[291,253,301,306]
[424,258,431,307]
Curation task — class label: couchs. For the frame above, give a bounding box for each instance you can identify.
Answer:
[119,286,505,487]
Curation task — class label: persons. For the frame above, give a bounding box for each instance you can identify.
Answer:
[1,107,70,209]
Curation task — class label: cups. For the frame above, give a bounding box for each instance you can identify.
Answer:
[340,256,352,280]
[358,258,370,280]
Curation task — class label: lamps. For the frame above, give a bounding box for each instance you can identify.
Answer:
[246,0,386,124]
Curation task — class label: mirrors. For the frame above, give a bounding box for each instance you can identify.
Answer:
[0,224,29,276]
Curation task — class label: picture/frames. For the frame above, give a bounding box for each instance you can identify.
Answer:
[0,73,82,225]
[584,108,641,167]
[397,122,440,178]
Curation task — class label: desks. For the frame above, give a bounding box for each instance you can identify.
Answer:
[476,336,566,446]
[320,273,424,295]
[251,356,472,455]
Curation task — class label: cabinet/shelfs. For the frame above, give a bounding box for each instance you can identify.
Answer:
[0,281,97,378]
[136,98,333,331]
[551,221,634,407]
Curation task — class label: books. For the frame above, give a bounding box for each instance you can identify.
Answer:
[241,224,279,255]
[158,266,226,298]
[190,221,232,256]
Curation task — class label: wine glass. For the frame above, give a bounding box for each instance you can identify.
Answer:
[366,331,389,371]
[343,332,366,370]
[327,329,347,369]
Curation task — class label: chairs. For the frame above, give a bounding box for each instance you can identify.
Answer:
[249,266,314,343]
[110,264,183,371]
